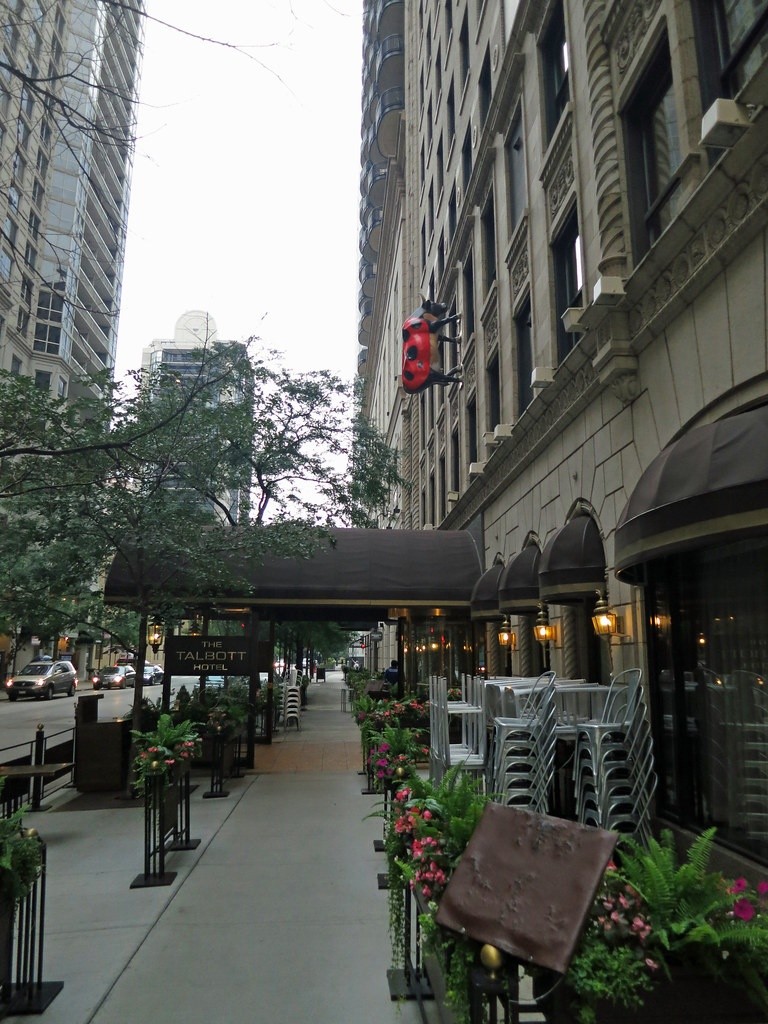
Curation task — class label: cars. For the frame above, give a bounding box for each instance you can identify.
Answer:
[92,666,136,690]
[115,658,152,668]
[143,664,165,686]
[193,676,224,692]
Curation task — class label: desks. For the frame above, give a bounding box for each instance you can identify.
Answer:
[0,762,73,823]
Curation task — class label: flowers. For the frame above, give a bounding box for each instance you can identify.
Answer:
[129,683,256,800]
[355,693,425,795]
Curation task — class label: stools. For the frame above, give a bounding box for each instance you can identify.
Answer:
[340,689,354,712]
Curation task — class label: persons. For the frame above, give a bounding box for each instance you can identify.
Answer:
[314,660,316,672]
[385,660,397,685]
[352,659,359,668]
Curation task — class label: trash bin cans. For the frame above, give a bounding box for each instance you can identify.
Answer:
[71,692,133,791]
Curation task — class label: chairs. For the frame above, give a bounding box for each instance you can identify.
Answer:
[696,668,768,846]
[259,670,304,733]
[426,668,660,859]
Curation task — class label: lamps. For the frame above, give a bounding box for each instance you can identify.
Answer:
[591,588,631,642]
[561,307,585,334]
[532,606,556,651]
[530,366,555,390]
[697,97,759,153]
[448,491,460,502]
[423,524,434,531]
[429,637,440,650]
[468,461,486,477]
[494,423,515,441]
[483,432,496,446]
[497,615,516,647]
[403,638,427,654]
[591,276,626,308]
[186,621,202,636]
[149,615,164,655]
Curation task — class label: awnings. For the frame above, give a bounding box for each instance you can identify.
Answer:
[471,562,509,621]
[498,545,547,616]
[613,401,768,587]
[539,517,608,606]
[103,525,483,719]
[40,623,79,638]
[78,629,105,644]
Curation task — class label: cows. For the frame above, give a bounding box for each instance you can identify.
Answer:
[401,292,463,394]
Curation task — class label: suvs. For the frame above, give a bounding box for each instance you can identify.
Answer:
[5,660,78,702]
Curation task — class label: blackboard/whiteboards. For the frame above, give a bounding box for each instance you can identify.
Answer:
[317,668,325,679]
